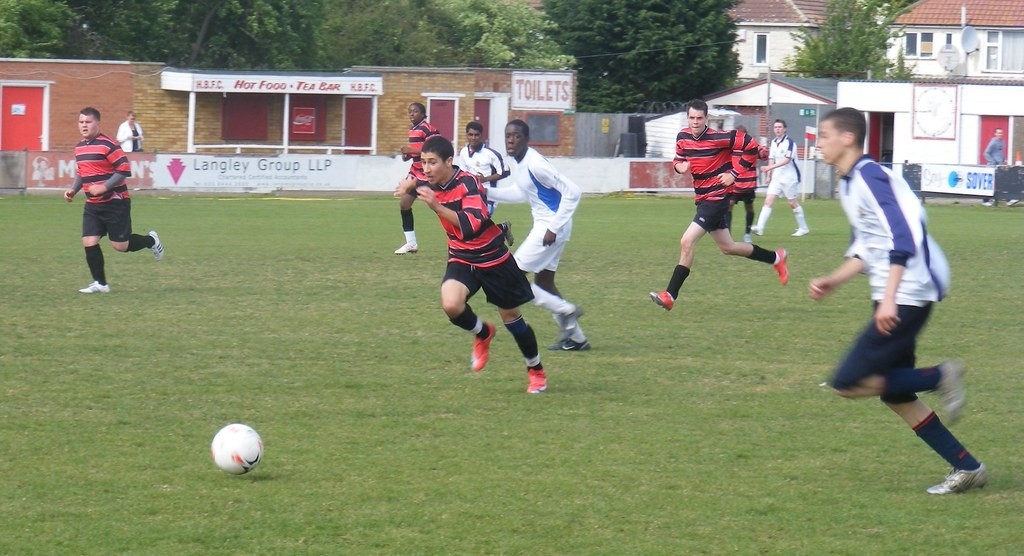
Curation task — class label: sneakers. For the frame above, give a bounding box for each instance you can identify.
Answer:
[750,225,763,236]
[774,248,788,284]
[526,367,548,393]
[558,306,582,344]
[78,281,110,293]
[936,359,966,422]
[926,462,987,494]
[148,231,164,259]
[395,243,418,255]
[548,338,590,351]
[791,227,810,237]
[504,220,514,246]
[649,290,674,311]
[472,323,494,371]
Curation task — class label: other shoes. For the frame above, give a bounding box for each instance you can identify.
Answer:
[744,232,753,243]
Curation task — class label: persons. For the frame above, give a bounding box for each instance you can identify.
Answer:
[116,110,145,191]
[809,107,987,496]
[64,107,163,294]
[727,119,810,244]
[393,102,591,394]
[982,128,1020,206]
[650,100,789,311]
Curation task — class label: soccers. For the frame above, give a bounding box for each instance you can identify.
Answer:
[212,424,263,475]
